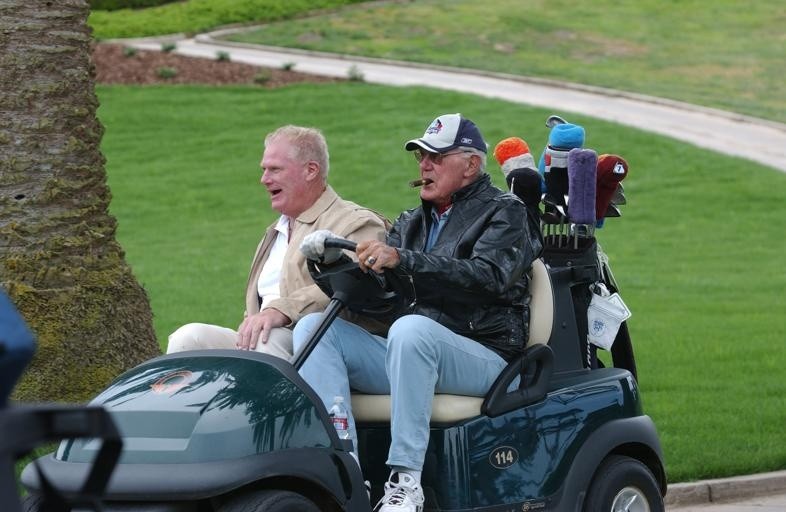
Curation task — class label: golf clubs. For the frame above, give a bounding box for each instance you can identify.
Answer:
[494,115,627,248]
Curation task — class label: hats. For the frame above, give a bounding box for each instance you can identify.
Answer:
[404,112,488,153]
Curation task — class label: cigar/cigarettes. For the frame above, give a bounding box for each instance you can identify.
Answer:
[408,178,433,187]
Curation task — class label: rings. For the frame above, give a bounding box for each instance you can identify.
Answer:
[367,256,377,265]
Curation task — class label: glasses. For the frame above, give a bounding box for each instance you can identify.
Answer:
[414,148,476,165]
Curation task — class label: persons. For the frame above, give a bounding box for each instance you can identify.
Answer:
[293,112,534,512]
[165,123,393,363]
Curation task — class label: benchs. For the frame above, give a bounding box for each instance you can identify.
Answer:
[334,258,555,425]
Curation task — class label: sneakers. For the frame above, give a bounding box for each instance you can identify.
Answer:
[378,473,426,512]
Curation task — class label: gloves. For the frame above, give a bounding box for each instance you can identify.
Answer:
[299,230,347,264]
